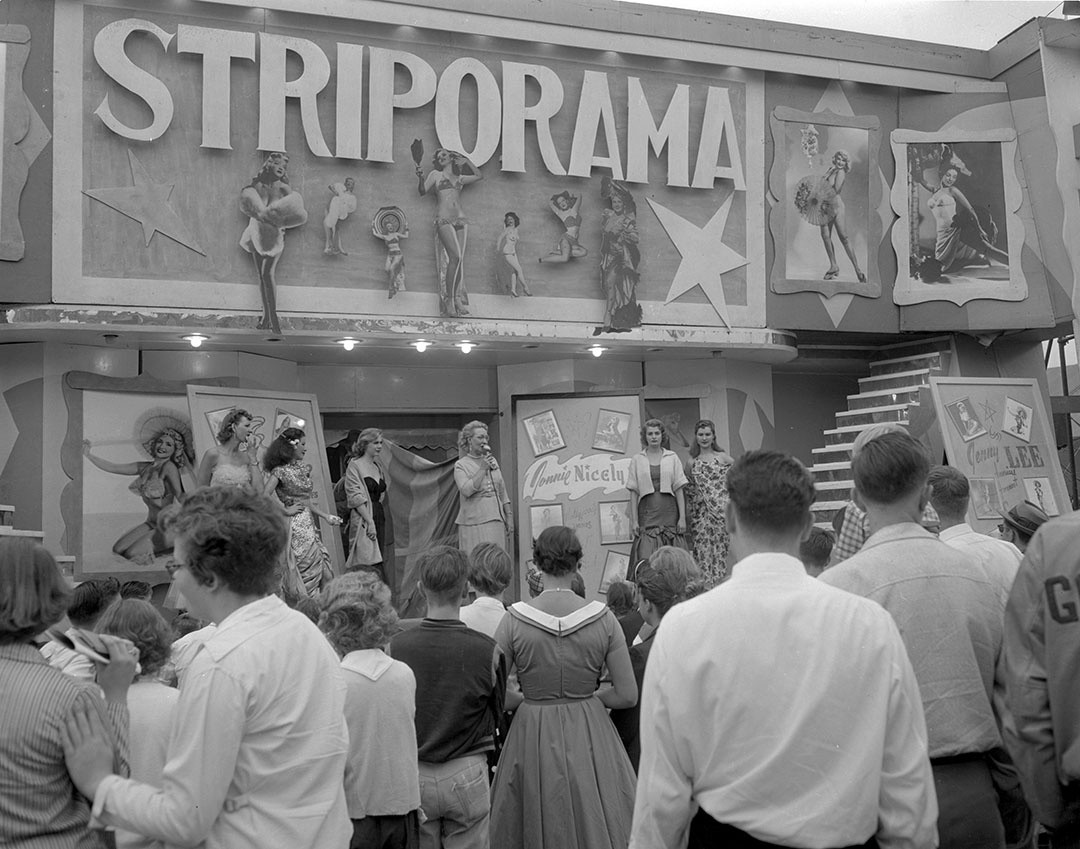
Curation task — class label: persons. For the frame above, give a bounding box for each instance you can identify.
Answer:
[627,419,690,562]
[684,420,738,588]
[239,147,587,339]
[198,408,265,496]
[344,427,387,569]
[956,402,1047,513]
[82,431,183,563]
[820,150,867,282]
[455,420,514,604]
[0,422,1080,849]
[263,428,344,598]
[918,164,1008,273]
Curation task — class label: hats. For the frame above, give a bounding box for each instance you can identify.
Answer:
[131,407,195,461]
[996,499,1050,537]
[525,569,543,593]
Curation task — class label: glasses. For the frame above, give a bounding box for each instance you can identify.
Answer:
[165,559,186,576]
[998,523,1009,532]
[476,433,490,441]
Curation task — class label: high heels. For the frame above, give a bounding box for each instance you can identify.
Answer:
[523,288,533,296]
[510,290,519,299]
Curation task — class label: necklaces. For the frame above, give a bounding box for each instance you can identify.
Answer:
[540,588,574,595]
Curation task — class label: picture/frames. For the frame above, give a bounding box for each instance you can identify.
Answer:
[598,499,635,546]
[520,408,567,458]
[592,407,633,454]
[598,549,630,594]
[969,476,1004,520]
[1022,476,1060,516]
[1001,395,1032,442]
[529,502,564,549]
[945,396,988,442]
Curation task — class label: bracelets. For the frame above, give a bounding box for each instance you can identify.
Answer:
[506,511,513,514]
[250,461,259,466]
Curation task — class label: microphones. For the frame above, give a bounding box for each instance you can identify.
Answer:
[483,444,492,472]
